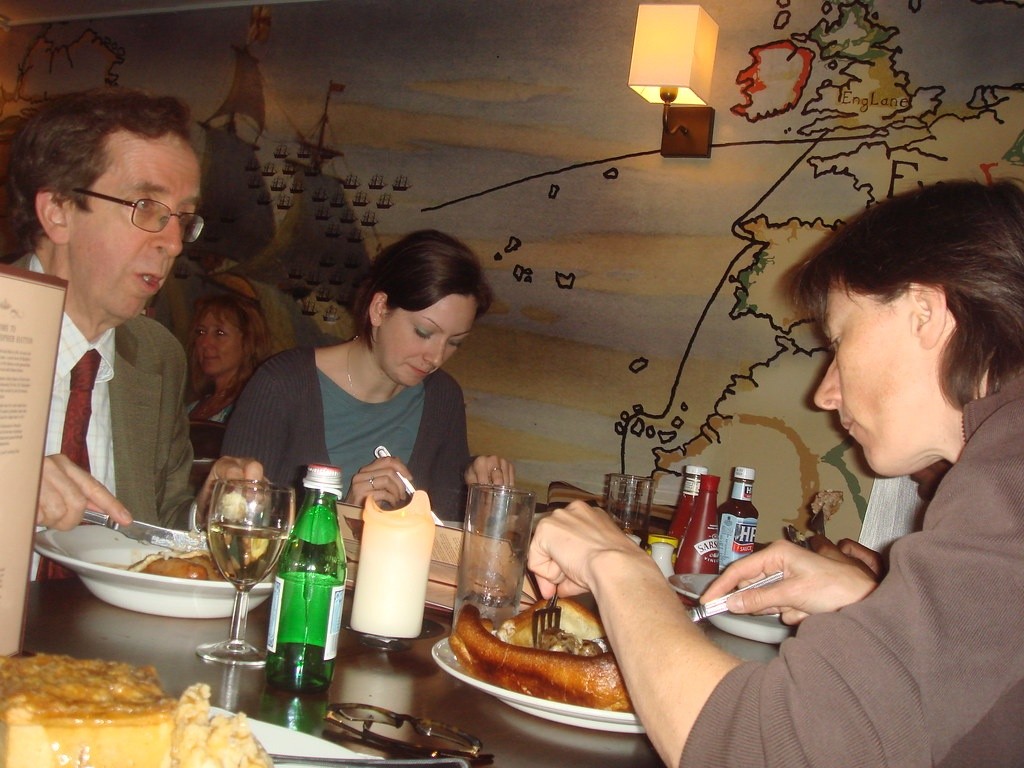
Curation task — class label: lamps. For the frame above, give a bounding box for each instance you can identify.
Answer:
[627,3,721,158]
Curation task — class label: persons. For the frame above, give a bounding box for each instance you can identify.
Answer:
[526,180,1024,768]
[0,84,271,582]
[217,229,516,532]
[177,285,270,425]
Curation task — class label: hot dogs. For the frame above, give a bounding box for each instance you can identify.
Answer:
[447,593,637,712]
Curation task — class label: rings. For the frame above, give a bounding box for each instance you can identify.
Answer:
[489,467,502,476]
[368,477,376,489]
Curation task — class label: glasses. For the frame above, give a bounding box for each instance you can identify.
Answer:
[73,188,204,243]
[325,705,483,761]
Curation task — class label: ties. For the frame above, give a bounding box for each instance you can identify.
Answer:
[37,349,101,580]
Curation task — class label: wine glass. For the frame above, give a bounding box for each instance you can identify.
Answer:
[194,479,292,666]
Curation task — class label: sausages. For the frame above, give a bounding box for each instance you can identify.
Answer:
[141,555,208,580]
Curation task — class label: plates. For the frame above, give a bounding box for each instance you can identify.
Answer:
[32,522,275,618]
[431,631,647,734]
[668,574,798,646]
[208,707,357,767]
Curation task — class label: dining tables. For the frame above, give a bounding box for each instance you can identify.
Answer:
[22,576,779,768]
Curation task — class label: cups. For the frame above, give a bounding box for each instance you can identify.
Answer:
[452,484,537,635]
[608,473,655,552]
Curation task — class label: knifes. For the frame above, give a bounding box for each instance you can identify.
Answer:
[688,572,784,623]
[83,507,208,550]
[787,524,809,550]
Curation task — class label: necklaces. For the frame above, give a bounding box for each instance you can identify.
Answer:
[346,335,395,403]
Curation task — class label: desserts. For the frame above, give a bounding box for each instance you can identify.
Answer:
[0,652,174,768]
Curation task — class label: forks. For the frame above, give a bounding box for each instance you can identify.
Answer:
[811,510,824,537]
[532,584,561,647]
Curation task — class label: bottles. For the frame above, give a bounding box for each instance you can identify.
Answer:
[676,474,722,605]
[262,463,347,696]
[716,466,758,574]
[646,532,678,579]
[668,464,708,543]
[349,490,435,639]
